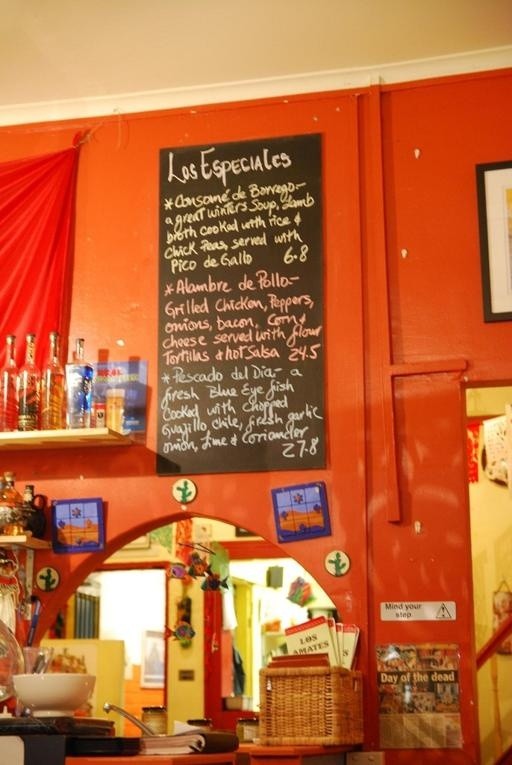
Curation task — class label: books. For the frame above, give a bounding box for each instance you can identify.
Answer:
[67,737,141,756]
[138,732,240,755]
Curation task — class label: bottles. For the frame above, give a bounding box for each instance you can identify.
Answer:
[236,718,259,743]
[0,471,45,538]
[0,330,92,431]
[92,348,152,439]
[139,706,167,737]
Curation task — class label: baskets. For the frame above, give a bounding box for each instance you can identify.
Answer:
[257,665,367,748]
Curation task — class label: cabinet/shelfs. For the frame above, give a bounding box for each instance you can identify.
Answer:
[1,429,132,550]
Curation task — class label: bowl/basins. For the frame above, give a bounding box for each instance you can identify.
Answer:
[226,696,243,711]
[13,674,97,718]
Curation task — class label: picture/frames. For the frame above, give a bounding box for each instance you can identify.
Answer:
[475,158,512,323]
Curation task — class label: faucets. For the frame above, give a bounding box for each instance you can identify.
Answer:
[103,703,158,739]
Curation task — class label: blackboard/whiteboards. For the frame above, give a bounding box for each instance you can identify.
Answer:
[156,133,327,475]
